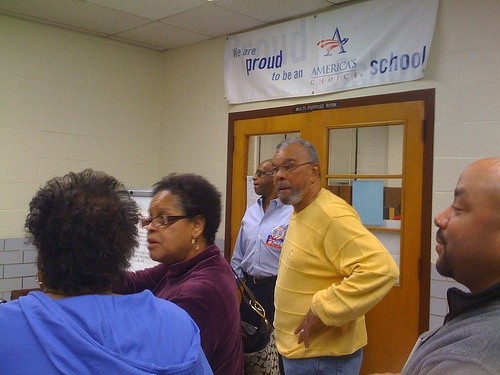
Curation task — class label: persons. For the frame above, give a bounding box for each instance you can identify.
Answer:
[270,138,400,375]
[229,159,294,375]
[0,168,213,375]
[402,156,500,375]
[111,173,244,375]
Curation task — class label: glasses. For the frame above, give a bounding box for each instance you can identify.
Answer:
[141,215,190,230]
[254,171,272,176]
[270,161,313,174]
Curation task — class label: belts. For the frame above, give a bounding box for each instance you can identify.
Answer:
[242,272,277,286]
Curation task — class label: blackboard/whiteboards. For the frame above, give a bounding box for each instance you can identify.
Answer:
[122,190,223,273]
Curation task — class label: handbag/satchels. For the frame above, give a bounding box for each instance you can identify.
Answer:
[230,264,281,375]
